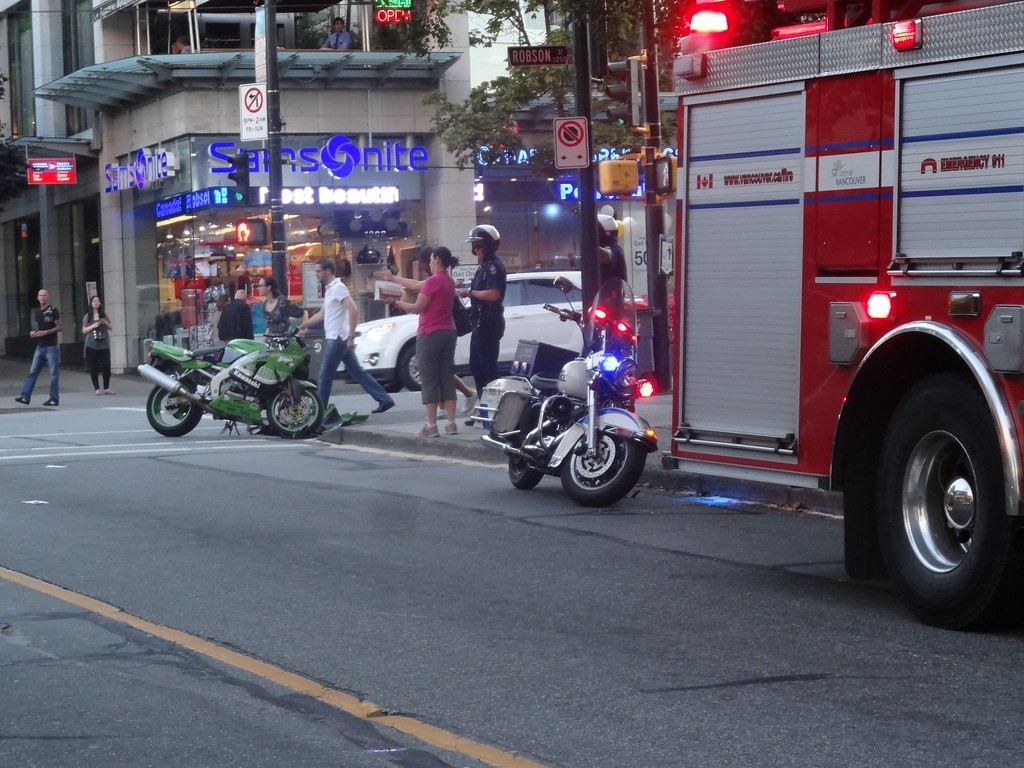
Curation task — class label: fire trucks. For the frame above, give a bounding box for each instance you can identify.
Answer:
[661,1,1024,628]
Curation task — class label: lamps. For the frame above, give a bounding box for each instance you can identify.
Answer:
[166,226,174,239]
[354,206,362,218]
[184,223,192,234]
[199,221,206,231]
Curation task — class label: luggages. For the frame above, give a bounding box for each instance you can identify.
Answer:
[139,328,153,363]
[157,298,181,341]
[365,295,385,322]
[180,288,207,329]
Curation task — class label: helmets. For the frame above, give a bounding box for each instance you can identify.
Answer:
[598,214,618,230]
[465,225,501,245]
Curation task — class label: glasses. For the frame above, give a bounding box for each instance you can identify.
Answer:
[258,285,267,287]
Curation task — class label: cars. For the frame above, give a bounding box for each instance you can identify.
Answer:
[335,271,583,393]
[136,284,176,322]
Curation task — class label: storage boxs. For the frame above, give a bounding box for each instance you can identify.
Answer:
[479,378,531,428]
[510,339,580,378]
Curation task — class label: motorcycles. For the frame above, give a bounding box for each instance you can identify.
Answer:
[470,276,659,507]
[137,328,323,439]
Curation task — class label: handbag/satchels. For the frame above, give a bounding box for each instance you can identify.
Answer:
[365,272,376,290]
[452,294,482,336]
[388,246,400,275]
[93,330,105,340]
[357,243,379,264]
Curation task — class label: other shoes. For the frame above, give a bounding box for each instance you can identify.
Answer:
[371,400,395,413]
[445,423,457,435]
[15,397,29,404]
[96,390,100,394]
[462,391,478,413]
[43,399,58,406]
[416,423,440,437]
[425,409,447,420]
[104,389,115,394]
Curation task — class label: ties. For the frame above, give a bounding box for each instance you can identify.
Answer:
[335,34,338,48]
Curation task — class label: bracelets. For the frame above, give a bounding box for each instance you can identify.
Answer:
[393,298,398,304]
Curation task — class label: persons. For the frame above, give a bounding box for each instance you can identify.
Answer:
[15,290,62,406]
[291,258,395,414]
[258,277,308,345]
[373,224,507,437]
[82,296,115,395]
[597,213,627,323]
[210,290,254,347]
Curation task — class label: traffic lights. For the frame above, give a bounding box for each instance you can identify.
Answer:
[606,59,645,126]
[227,153,249,200]
[236,219,267,246]
[653,156,676,195]
